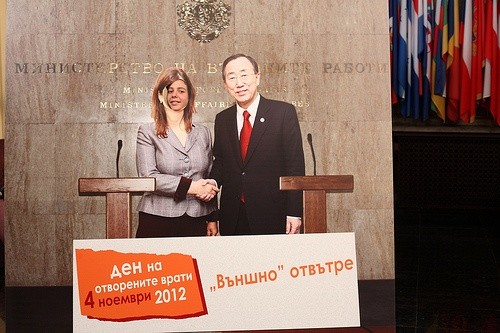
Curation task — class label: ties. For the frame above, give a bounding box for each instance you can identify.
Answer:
[239,111,252,160]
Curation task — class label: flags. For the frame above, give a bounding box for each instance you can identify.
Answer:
[387,0,500,124]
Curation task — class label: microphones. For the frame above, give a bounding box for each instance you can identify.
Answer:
[307,133,317,175]
[115,140,122,177]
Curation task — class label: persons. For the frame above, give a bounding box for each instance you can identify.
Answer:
[195,54,304,237]
[166,80,189,110]
[136,66,219,238]
